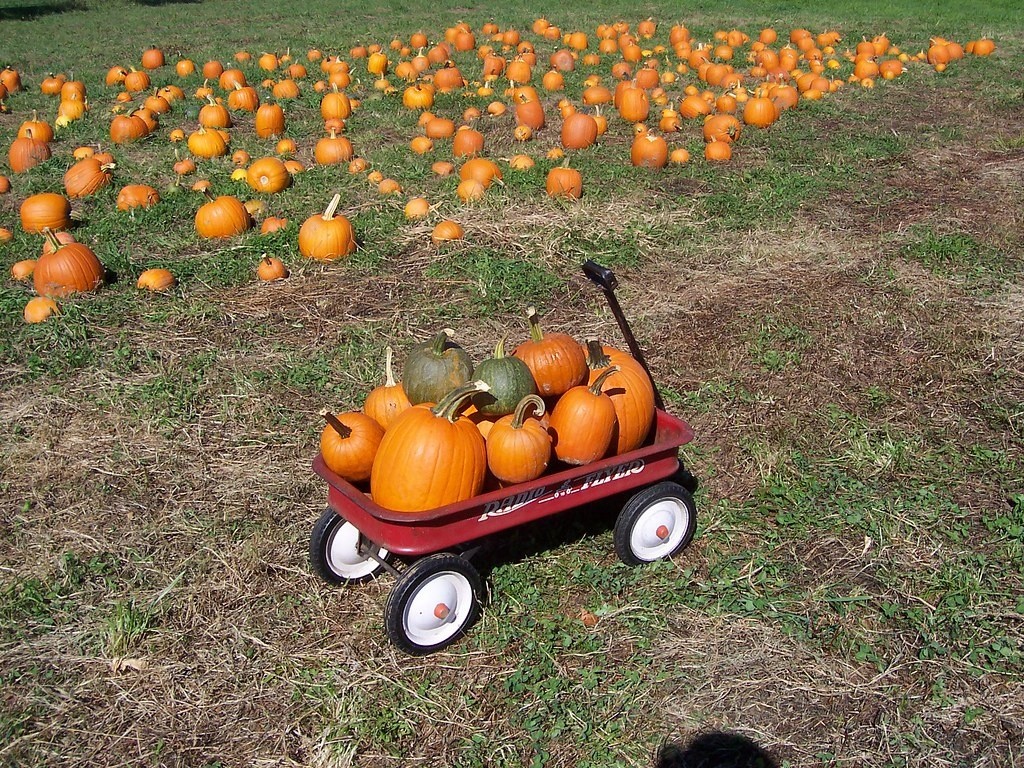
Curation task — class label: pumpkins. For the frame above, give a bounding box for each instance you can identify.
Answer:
[320,305,655,512]
[0,16,995,322]
